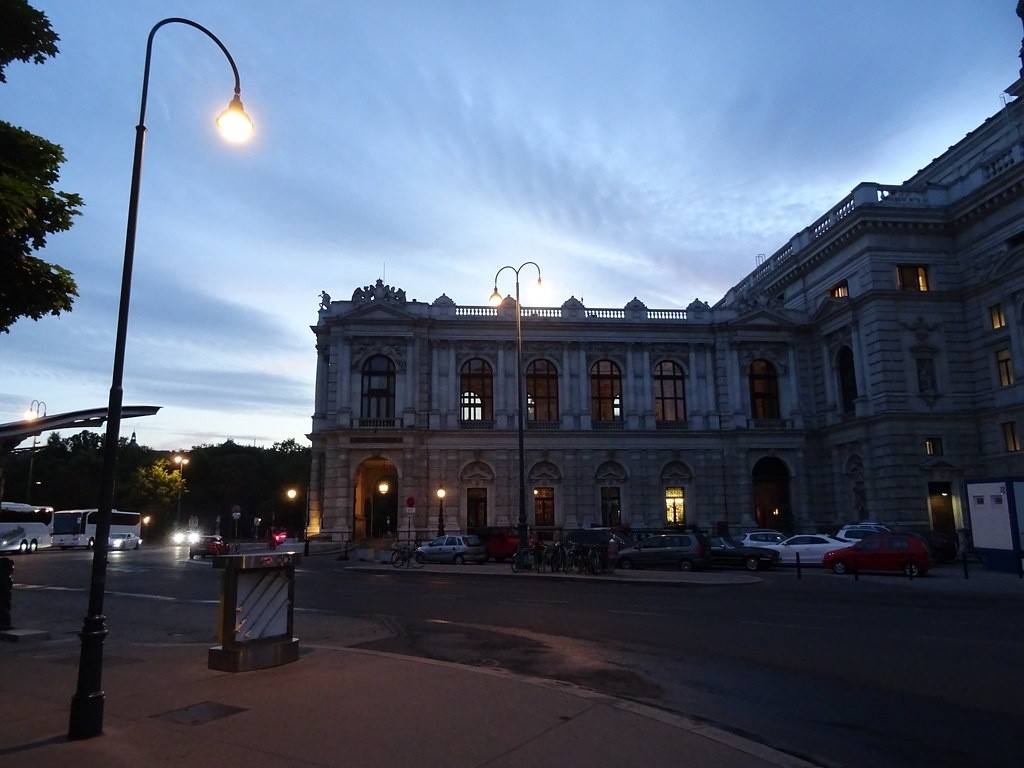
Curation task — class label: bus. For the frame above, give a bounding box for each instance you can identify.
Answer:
[52,508,142,550]
[0,502,54,553]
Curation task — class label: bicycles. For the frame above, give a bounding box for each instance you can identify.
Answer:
[509,536,616,577]
[391,543,425,567]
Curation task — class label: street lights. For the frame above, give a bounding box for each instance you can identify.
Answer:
[68,15,256,740]
[25,400,47,503]
[173,455,188,530]
[437,485,446,538]
[490,261,546,568]
[378,482,388,539]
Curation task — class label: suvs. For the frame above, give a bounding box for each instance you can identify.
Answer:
[837,523,890,545]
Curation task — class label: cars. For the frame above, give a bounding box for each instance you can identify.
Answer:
[822,533,935,577]
[742,531,788,551]
[190,536,224,558]
[706,535,778,570]
[108,533,143,551]
[618,533,712,571]
[773,533,855,568]
[416,535,485,564]
